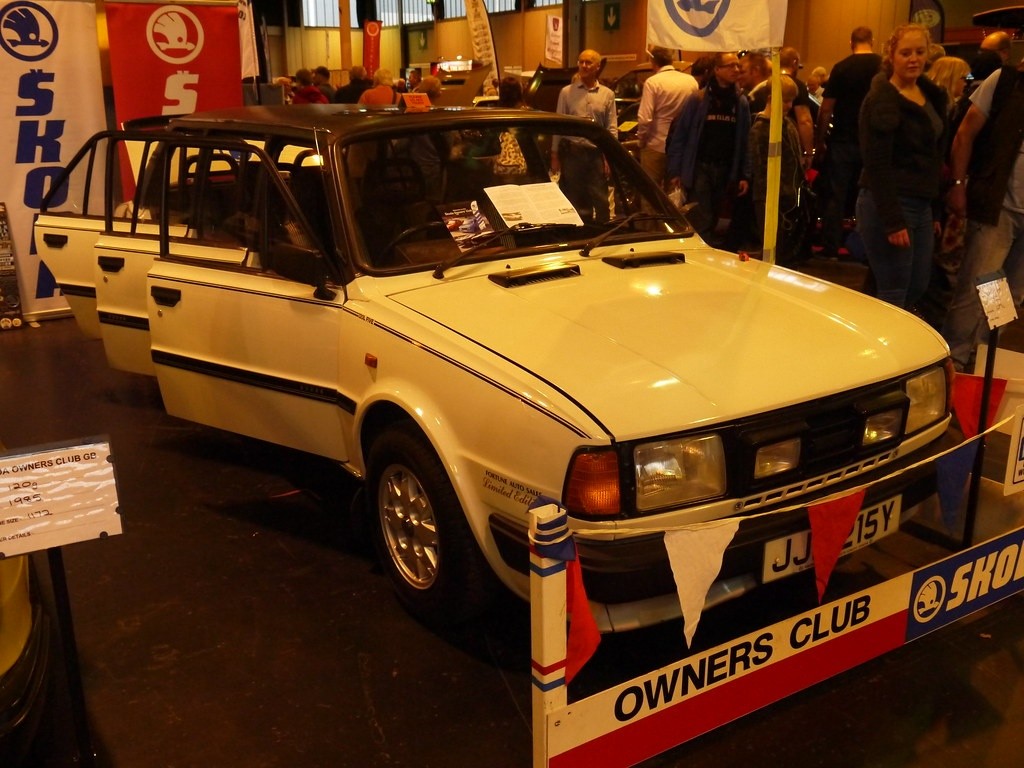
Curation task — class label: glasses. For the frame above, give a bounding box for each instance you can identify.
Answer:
[717,62,743,71]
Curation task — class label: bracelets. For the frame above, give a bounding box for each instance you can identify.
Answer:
[952,179,964,184]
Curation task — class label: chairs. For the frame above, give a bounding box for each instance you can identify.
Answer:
[177,154,426,242]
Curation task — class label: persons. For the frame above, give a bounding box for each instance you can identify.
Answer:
[273,22,1024,375]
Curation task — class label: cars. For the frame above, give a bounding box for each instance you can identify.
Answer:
[35,112,959,632]
